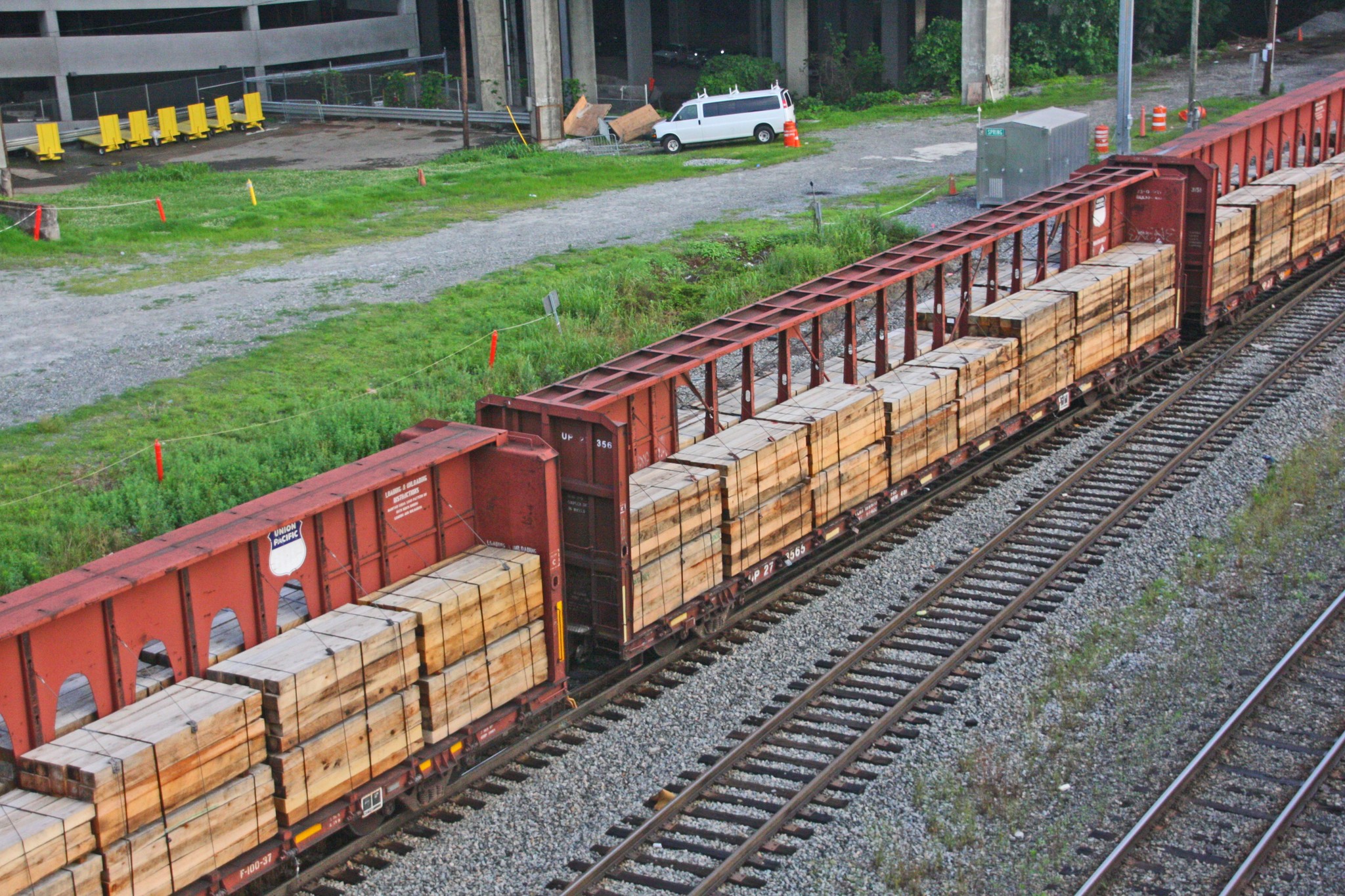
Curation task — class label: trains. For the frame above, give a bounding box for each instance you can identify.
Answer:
[0,67,1343,896]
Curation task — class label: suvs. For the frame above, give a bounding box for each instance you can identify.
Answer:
[371,95,385,109]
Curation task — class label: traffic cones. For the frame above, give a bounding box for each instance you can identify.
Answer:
[793,127,801,148]
[1297,27,1302,42]
[945,172,959,197]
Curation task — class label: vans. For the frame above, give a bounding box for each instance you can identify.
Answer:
[649,86,797,155]
